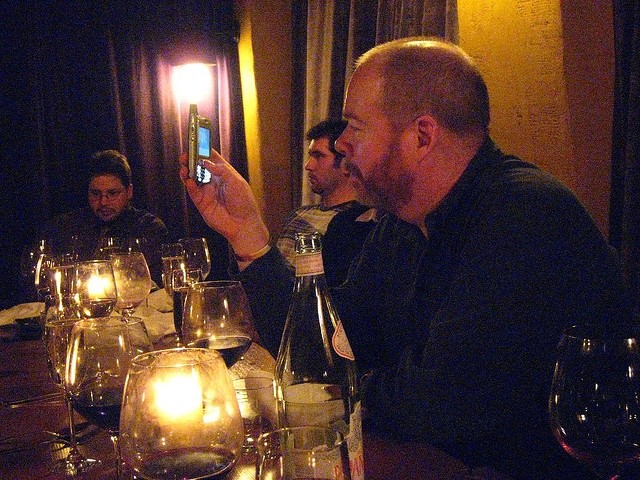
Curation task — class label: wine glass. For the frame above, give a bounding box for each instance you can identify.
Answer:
[42,265,75,306]
[548,326,640,480]
[68,260,118,320]
[172,267,201,339]
[181,280,255,368]
[42,320,100,476]
[110,251,152,315]
[118,348,243,480]
[159,242,191,310]
[64,316,155,479]
[177,237,210,284]
[97,235,124,261]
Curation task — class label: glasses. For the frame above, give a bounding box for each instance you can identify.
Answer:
[87,187,125,200]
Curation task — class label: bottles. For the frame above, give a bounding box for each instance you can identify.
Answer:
[274,230,365,480]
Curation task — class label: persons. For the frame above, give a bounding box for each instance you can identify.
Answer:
[21,149,170,282]
[272,119,384,290]
[179,36,629,468]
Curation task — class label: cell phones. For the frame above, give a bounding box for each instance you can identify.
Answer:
[187,104,213,184]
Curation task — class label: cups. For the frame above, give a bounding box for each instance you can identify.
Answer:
[43,294,82,359]
[256,425,343,479]
[234,373,278,461]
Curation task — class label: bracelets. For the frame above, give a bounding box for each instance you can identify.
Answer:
[234,235,276,263]
